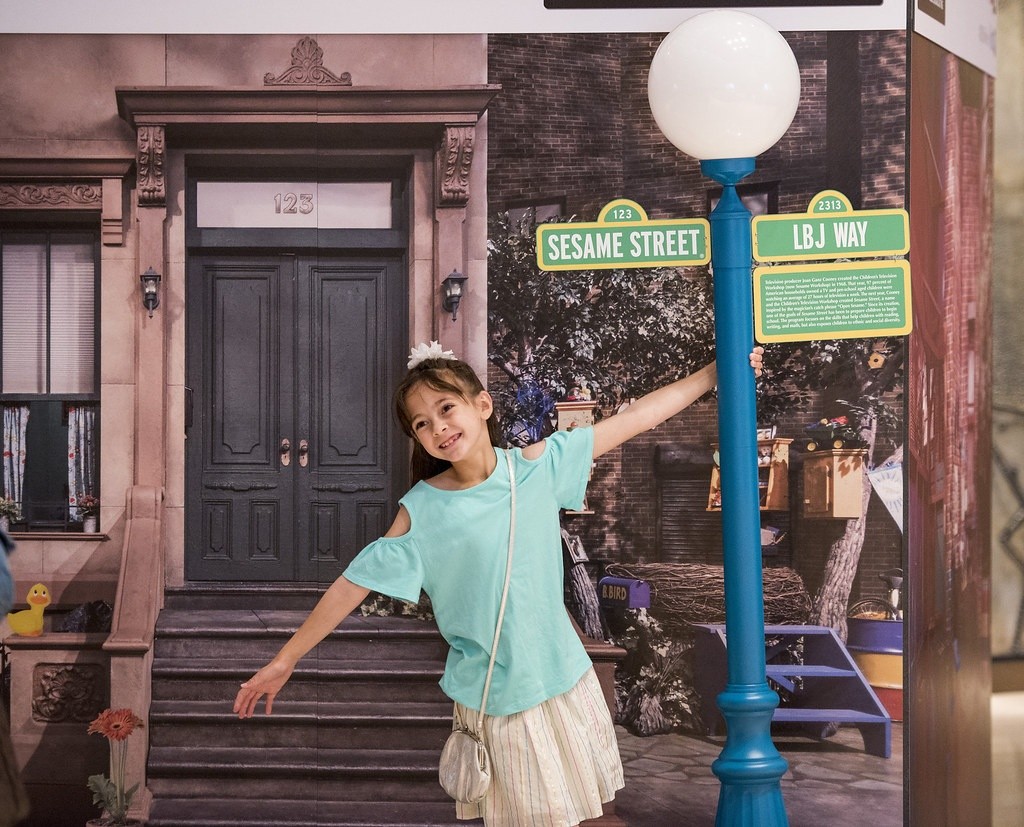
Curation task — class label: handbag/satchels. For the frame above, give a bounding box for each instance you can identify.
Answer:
[438,726,491,803]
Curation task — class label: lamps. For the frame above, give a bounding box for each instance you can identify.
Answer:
[139,266,162,320]
[441,268,469,323]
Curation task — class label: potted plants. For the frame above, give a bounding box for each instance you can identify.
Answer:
[0,497,25,532]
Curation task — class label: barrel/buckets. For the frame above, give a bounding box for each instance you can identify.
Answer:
[847,610,904,722]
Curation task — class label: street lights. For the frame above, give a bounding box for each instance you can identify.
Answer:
[646,11,803,825]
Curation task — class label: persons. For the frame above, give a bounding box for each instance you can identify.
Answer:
[233,341,764,827]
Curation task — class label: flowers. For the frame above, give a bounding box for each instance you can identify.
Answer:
[86,708,145,827]
[75,495,100,516]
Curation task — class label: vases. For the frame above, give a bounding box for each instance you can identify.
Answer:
[82,515,96,533]
[86,818,144,827]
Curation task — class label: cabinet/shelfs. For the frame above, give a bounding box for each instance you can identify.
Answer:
[706,438,795,512]
